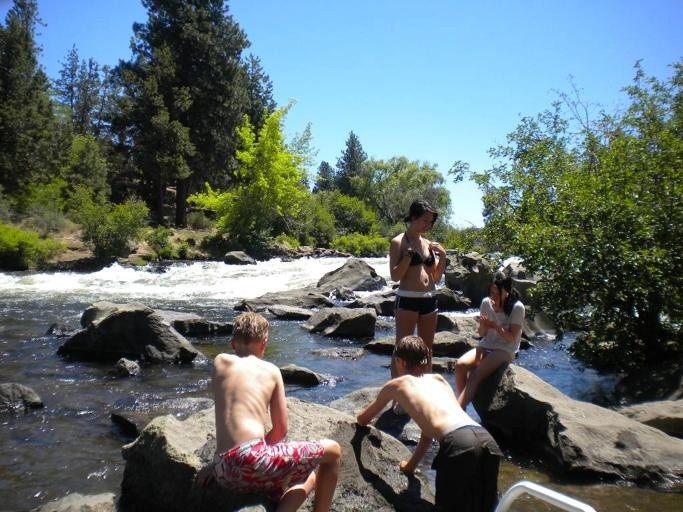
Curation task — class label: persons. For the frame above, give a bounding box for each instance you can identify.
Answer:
[210,311,341,511]
[454,275,524,411]
[389,199,448,415]
[355,333,503,512]
